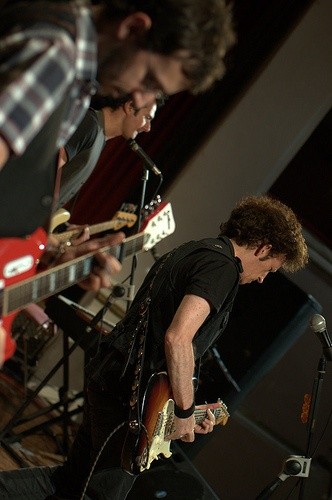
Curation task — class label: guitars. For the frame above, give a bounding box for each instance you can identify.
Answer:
[0,195,176,367]
[121,372,230,475]
[50,203,138,246]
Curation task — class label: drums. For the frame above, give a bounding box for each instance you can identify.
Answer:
[11,304,57,359]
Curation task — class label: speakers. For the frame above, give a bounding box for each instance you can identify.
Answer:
[124,441,220,500]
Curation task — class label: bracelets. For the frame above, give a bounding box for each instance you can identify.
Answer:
[174,401,196,419]
[47,240,71,268]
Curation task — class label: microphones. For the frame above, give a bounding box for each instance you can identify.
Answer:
[309,314,332,362]
[128,138,161,175]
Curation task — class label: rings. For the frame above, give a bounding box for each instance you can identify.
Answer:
[179,435,184,438]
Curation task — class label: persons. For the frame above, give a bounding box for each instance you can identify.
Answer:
[0,0,236,291]
[0,193,308,500]
[56,96,164,208]
